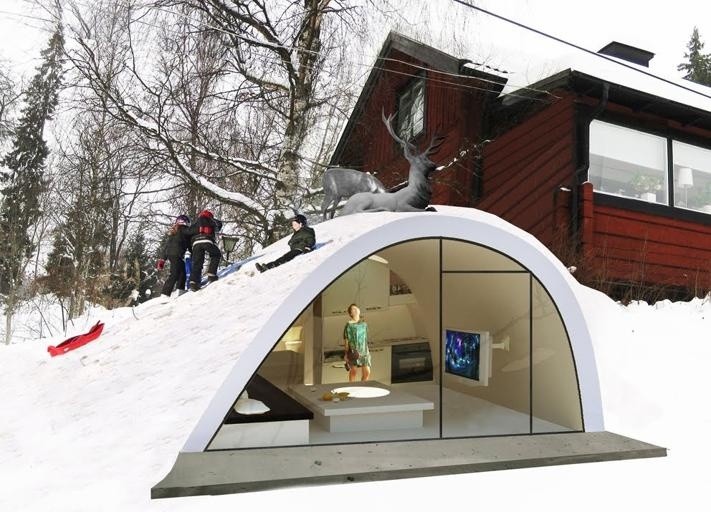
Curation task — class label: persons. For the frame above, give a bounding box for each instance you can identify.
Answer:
[189,211,223,292]
[343,303,371,383]
[157,215,213,303]
[254,214,315,272]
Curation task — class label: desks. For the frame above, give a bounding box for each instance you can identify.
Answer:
[285,379,436,434]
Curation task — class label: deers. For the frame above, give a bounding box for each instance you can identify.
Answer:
[321,168,389,222]
[339,105,451,217]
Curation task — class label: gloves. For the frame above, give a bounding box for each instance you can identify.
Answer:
[199,227,213,234]
[158,260,165,269]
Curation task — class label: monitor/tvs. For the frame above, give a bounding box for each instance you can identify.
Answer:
[442,326,493,387]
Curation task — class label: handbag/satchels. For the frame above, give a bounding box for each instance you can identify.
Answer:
[347,348,359,360]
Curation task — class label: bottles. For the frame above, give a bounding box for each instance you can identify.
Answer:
[391,283,408,294]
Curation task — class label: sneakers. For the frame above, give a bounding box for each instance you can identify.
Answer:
[262,264,270,271]
[160,294,170,303]
[208,275,217,281]
[189,284,199,292]
[255,263,263,274]
[174,290,186,298]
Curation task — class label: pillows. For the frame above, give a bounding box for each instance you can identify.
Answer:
[232,387,272,415]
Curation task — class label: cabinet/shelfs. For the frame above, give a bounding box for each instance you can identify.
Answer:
[313,258,391,320]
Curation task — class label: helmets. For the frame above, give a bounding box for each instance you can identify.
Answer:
[177,215,191,226]
[198,210,213,218]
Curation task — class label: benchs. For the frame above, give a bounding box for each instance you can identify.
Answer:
[214,369,314,445]
